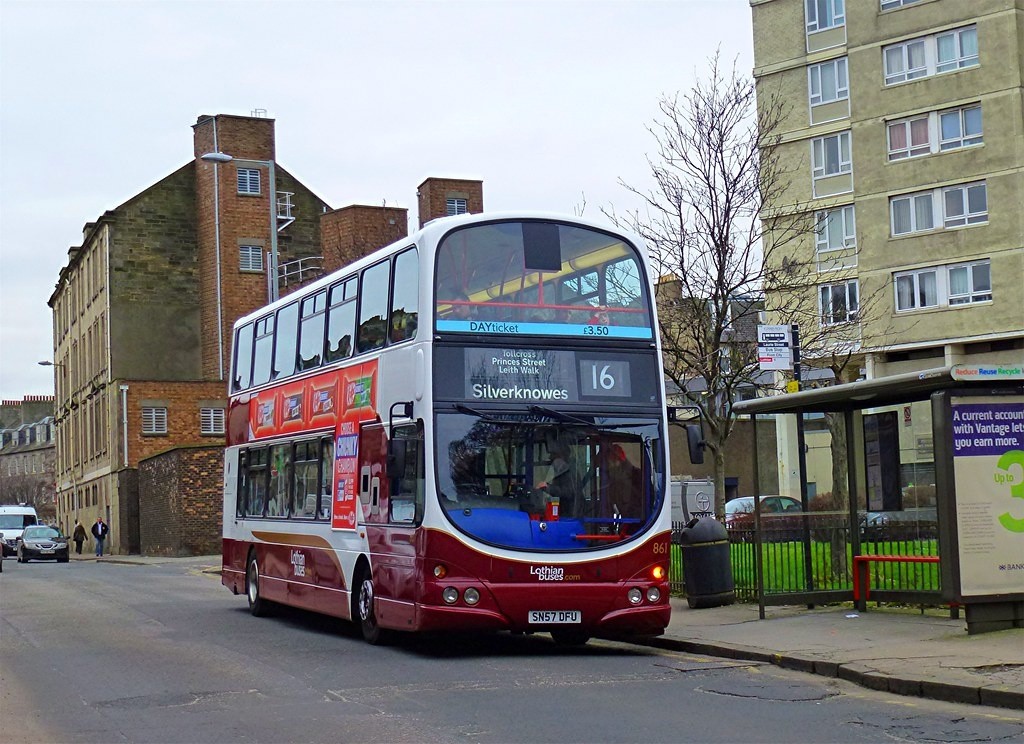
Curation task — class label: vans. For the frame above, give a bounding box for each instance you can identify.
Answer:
[0,502,40,556]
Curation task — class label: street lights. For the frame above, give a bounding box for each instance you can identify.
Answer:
[202,151,280,301]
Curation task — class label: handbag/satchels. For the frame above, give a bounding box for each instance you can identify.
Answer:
[72,527,79,541]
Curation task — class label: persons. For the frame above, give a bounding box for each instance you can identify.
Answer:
[605,445,642,535]
[73,522,88,554]
[452,293,475,321]
[91,517,108,556]
[536,446,588,527]
[588,305,618,326]
[552,304,572,323]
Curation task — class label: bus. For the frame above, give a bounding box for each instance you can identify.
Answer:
[219,210,710,646]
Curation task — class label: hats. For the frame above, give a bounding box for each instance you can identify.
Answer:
[605,444,626,461]
[549,443,571,460]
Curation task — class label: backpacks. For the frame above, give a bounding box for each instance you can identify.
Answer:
[76,527,83,539]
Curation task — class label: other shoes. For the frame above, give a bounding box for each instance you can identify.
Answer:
[100,554,102,556]
[96,554,99,556]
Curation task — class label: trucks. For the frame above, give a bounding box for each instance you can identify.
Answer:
[668,477,717,525]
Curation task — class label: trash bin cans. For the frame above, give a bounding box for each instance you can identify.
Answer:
[679,516,735,610]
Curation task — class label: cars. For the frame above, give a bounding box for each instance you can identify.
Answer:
[15,523,71,564]
[712,494,804,528]
